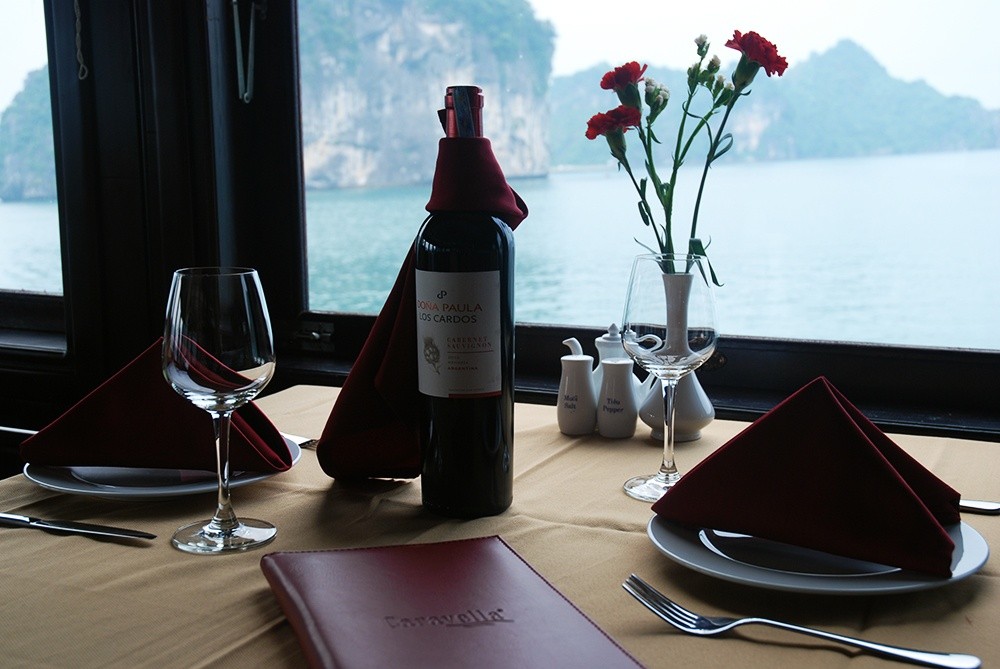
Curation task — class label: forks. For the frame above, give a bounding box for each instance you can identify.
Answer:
[298,439,320,450]
[621,572,983,669]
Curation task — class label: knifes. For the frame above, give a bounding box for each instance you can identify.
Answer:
[0,511,158,541]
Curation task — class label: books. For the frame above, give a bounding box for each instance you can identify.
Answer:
[260,533,647,669]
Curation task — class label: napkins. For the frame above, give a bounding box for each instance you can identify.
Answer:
[652,373,962,572]
[20,333,294,474]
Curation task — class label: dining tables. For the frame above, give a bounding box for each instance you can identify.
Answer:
[0,384,1000,669]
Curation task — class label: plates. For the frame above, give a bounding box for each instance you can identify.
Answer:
[645,513,989,597]
[23,436,303,503]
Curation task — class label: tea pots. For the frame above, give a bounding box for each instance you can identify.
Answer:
[562,322,656,416]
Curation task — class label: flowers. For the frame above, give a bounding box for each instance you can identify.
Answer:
[585,30,789,289]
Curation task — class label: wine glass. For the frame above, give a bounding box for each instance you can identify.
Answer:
[162,266,279,556]
[619,251,719,503]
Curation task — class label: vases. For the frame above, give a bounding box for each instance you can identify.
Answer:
[639,273,715,443]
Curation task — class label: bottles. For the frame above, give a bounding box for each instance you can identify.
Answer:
[415,86,516,519]
[596,358,639,439]
[556,355,599,435]
